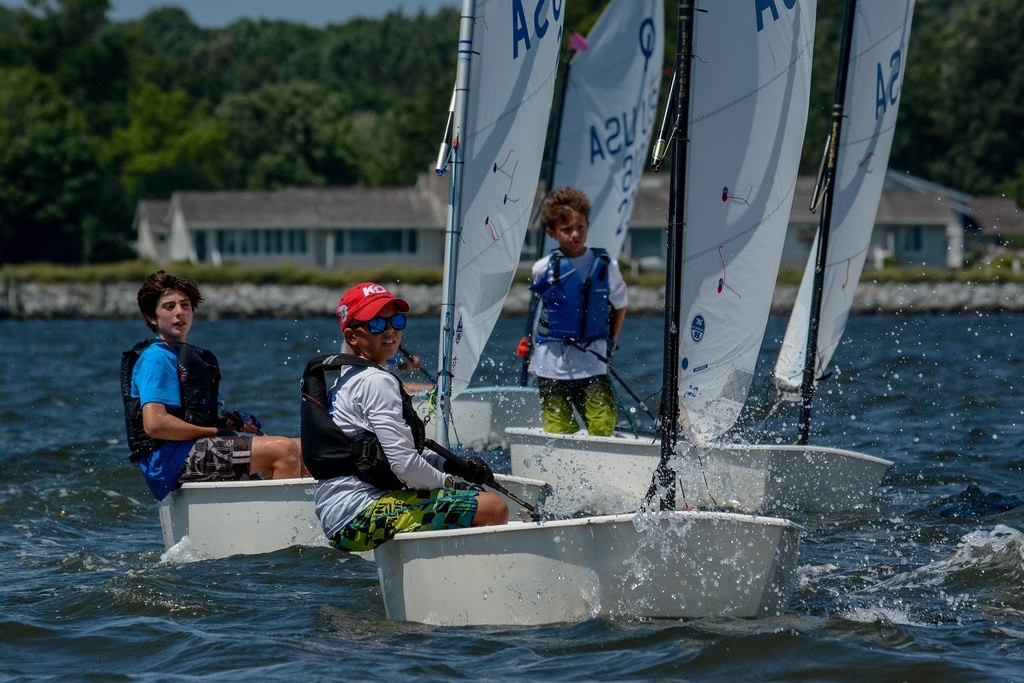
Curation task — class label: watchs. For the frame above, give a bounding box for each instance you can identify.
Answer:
[608,339,619,351]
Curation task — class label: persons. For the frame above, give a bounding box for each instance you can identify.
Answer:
[123,269,312,493]
[531,186,630,438]
[340,335,421,396]
[313,281,510,553]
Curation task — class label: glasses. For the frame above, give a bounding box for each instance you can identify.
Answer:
[348,314,407,334]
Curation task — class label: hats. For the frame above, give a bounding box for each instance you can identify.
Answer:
[336,282,411,329]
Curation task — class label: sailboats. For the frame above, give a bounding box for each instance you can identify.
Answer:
[155,0,921,627]
